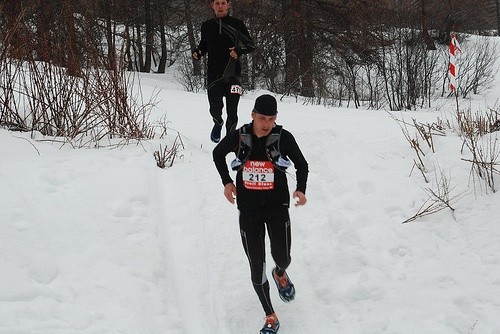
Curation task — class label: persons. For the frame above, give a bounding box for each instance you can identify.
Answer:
[212,94,309,334]
[192,0,255,142]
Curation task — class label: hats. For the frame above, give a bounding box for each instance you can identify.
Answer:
[254,94,278,116]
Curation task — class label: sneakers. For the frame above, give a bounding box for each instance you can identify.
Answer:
[210,124,222,143]
[271,267,295,301]
[260,316,280,334]
